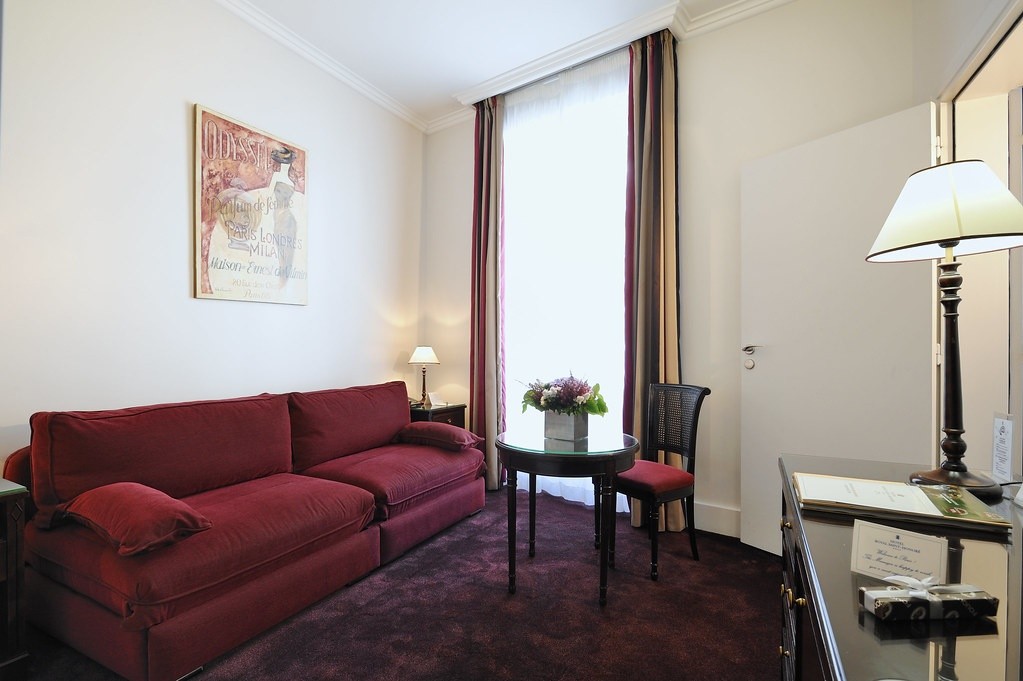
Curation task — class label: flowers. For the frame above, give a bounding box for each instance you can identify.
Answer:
[514,370,608,417]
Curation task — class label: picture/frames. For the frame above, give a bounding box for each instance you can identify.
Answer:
[195,103,309,307]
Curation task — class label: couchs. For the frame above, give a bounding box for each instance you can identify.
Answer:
[2,381,488,681]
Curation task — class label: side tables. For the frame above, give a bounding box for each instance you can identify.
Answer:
[410,404,467,429]
[0,477,31,668]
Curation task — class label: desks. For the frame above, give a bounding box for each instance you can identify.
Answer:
[495,429,640,607]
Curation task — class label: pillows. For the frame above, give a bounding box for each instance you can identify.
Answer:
[389,421,486,450]
[55,482,215,556]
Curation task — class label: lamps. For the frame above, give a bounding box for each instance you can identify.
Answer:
[865,159,1023,498]
[408,346,441,405]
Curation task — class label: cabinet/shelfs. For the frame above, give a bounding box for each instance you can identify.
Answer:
[777,453,1023,681]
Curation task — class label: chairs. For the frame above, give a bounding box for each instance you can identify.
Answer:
[603,383,711,581]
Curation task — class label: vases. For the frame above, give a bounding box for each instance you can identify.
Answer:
[544,410,588,440]
